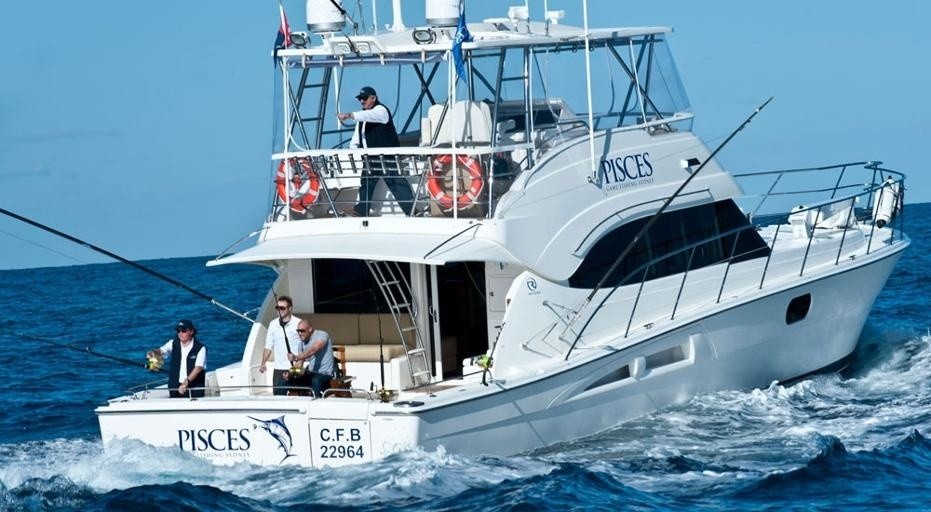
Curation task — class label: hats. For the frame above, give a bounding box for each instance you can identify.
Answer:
[174,319,193,329]
[355,86,376,97]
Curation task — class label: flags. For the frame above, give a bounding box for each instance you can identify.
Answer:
[452,1,473,84]
[273,10,291,69]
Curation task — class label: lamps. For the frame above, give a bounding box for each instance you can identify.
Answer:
[289,31,311,49]
[411,27,437,45]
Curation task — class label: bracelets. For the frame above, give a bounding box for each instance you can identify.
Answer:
[184,378,191,383]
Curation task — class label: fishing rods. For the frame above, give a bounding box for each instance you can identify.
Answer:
[0,331,175,378]
[262,268,303,387]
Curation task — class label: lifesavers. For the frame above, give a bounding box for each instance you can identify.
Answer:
[426,153,484,211]
[274,158,320,214]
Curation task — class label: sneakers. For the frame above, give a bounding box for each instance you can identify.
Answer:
[342,208,364,217]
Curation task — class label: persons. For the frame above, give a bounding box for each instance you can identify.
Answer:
[283,320,335,397]
[260,295,309,396]
[337,86,424,217]
[147,319,211,397]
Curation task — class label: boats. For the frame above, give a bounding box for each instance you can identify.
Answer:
[94,0,911,470]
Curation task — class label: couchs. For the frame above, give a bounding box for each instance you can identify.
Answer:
[265,312,428,391]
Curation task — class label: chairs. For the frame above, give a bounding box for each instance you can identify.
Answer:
[288,345,356,397]
